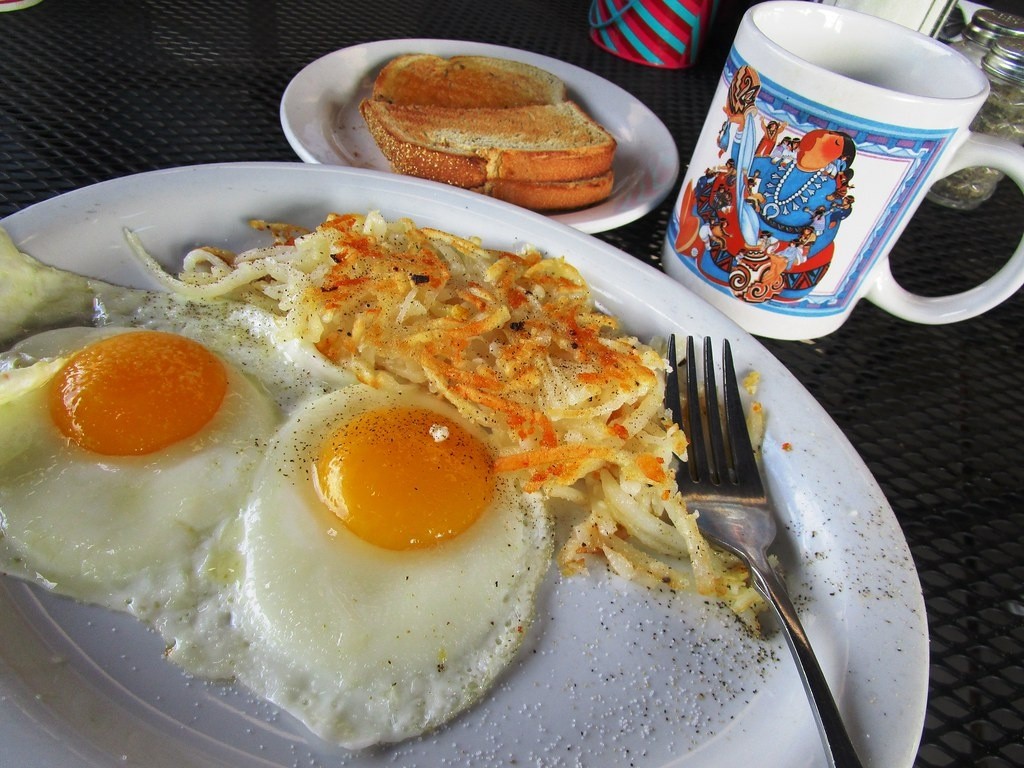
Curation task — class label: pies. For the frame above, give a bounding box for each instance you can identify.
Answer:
[119,204,777,630]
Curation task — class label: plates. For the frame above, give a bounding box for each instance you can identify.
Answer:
[0,160,929,768]
[278,38,679,234]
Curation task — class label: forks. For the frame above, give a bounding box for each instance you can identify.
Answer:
[664,334,863,768]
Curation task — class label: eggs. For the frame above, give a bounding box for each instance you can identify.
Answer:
[0,219,337,616]
[160,383,551,750]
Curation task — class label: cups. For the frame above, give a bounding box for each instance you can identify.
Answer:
[661,0,1024,340]
[588,0,719,69]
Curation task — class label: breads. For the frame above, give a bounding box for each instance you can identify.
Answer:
[357,53,617,212]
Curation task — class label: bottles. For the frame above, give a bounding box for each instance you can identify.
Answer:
[949,9,1024,71]
[925,40,1024,212]
